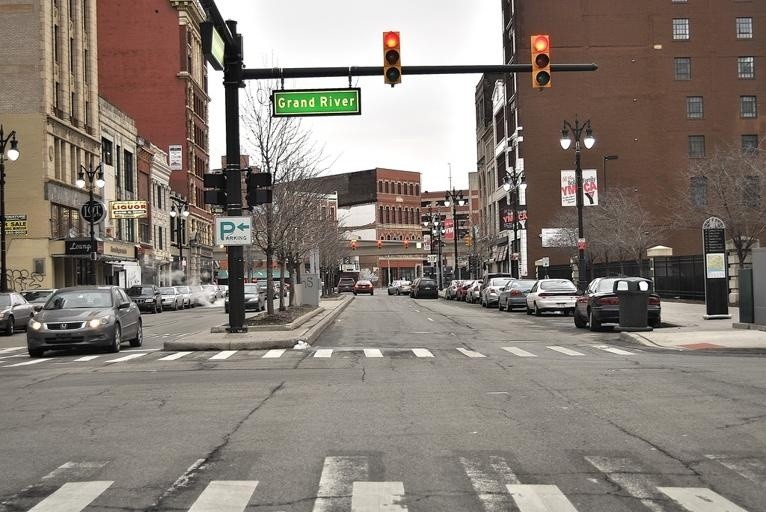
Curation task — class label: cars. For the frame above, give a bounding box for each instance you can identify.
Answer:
[575,275,661,330]
[23,289,57,312]
[128,284,229,313]
[388,280,412,296]
[257,280,290,299]
[527,278,578,315]
[353,280,373,296]
[445,273,517,309]
[27,285,143,358]
[408,278,420,297]
[497,279,538,311]
[0,292,35,334]
[224,283,267,312]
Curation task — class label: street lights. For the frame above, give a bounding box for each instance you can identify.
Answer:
[444,186,465,280]
[170,195,189,284]
[433,223,445,289]
[502,165,528,280]
[423,205,439,279]
[76,162,105,285]
[0,124,20,292]
[559,113,596,294]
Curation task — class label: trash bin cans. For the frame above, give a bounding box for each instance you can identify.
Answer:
[611,276,654,333]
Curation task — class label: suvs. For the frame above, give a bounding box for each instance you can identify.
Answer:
[337,278,355,294]
[412,278,438,299]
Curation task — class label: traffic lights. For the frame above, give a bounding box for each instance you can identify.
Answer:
[470,236,473,247]
[378,240,382,249]
[352,240,357,251]
[405,241,409,251]
[531,33,552,90]
[252,172,274,206]
[383,31,402,86]
[464,235,470,246]
[203,173,225,204]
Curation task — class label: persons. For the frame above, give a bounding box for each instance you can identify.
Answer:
[568,254,580,287]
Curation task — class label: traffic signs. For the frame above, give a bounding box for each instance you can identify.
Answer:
[274,90,360,114]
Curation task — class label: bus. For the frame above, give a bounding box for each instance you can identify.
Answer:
[423,255,452,287]
